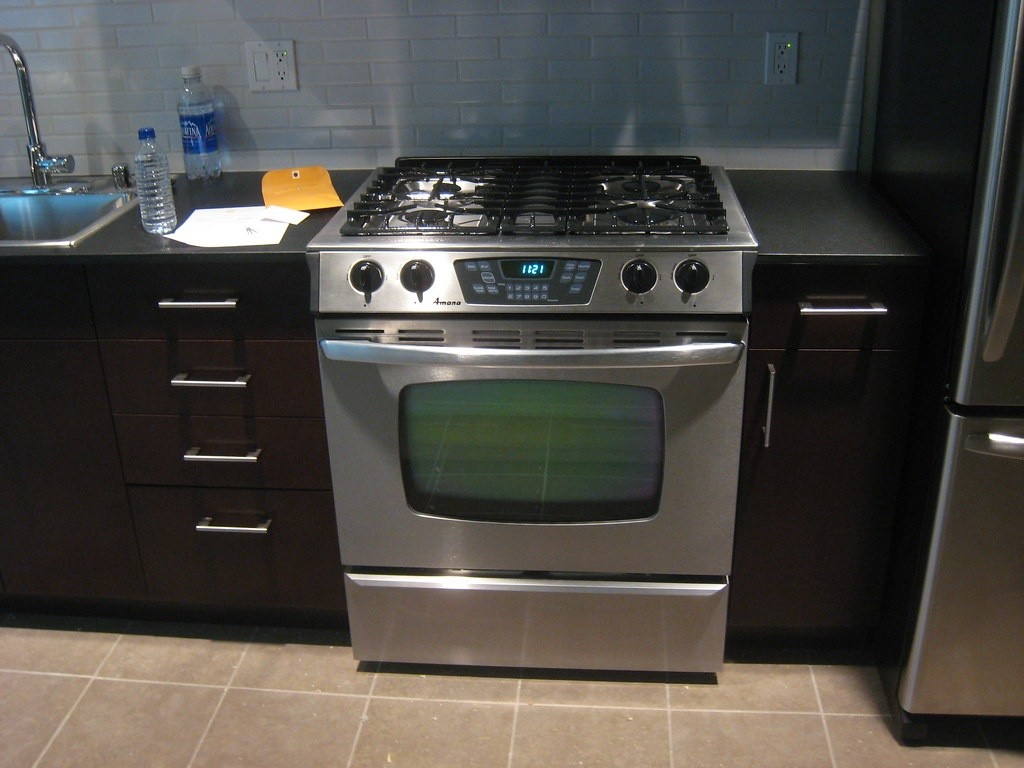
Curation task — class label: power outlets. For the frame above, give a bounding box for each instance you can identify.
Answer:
[764,30,801,86]
[246,38,299,92]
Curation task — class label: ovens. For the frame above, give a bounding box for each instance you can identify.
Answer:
[309,317,748,673]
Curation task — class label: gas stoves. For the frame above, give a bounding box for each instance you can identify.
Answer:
[313,150,757,252]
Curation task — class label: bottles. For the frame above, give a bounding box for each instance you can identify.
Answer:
[132,126,176,236]
[176,66,224,179]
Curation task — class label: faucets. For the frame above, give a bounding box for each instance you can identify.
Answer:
[0,31,52,188]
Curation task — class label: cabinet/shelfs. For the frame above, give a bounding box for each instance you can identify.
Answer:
[727,265,957,630]
[1,258,147,601]
[83,259,346,614]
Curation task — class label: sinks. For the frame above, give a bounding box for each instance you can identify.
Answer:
[0,184,147,248]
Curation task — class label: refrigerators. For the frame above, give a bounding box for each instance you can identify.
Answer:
[860,2,1024,721]
